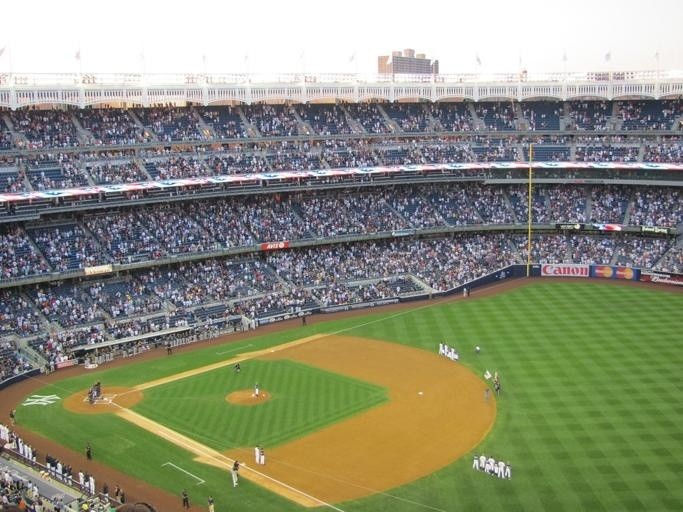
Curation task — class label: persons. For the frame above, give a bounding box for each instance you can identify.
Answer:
[0,97,682,512]
